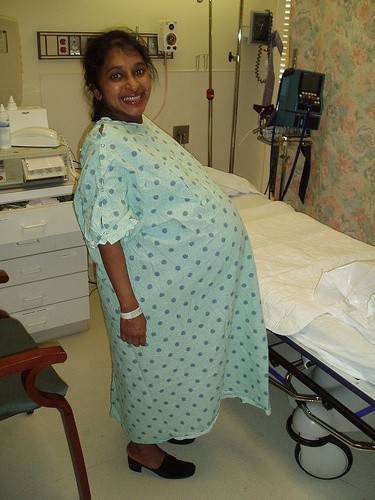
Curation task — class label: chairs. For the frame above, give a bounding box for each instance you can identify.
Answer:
[0,270,92,499]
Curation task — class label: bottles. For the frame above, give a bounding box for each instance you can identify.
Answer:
[6,95,17,110]
[0,104,12,149]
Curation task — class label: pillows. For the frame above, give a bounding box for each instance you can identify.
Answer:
[202,166,266,196]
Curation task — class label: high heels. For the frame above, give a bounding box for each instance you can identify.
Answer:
[168,437,196,445]
[128,451,196,479]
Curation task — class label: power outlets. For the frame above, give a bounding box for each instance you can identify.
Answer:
[173,126,189,144]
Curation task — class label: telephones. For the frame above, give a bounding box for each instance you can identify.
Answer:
[10,125,63,148]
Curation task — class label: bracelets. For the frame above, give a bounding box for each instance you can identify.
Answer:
[121,307,142,319]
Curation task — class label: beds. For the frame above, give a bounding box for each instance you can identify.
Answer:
[205,194,375,453]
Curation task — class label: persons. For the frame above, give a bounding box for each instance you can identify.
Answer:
[74,30,271,479]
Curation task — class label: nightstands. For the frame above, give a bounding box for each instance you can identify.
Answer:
[0,180,92,345]
